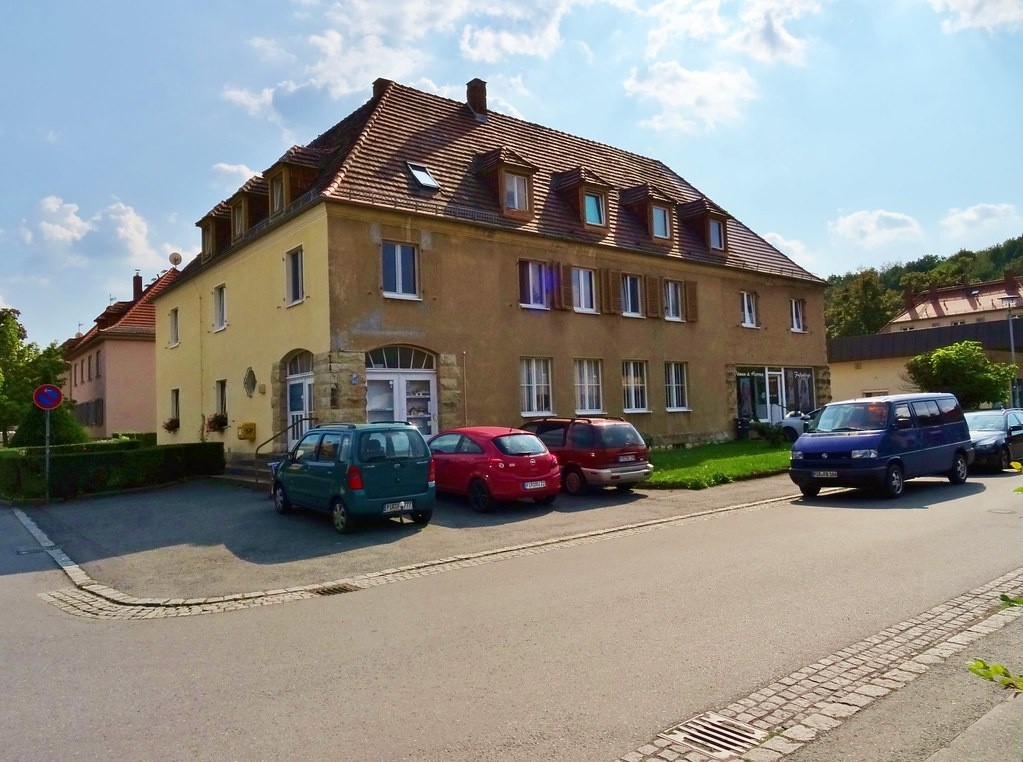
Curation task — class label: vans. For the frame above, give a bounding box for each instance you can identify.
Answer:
[790,392,976,499]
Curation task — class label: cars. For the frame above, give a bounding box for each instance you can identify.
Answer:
[271,420,435,533]
[517,415,655,495]
[782,407,827,441]
[961,407,1023,473]
[423,426,560,511]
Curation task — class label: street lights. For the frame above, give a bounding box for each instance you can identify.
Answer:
[998,296,1021,407]
